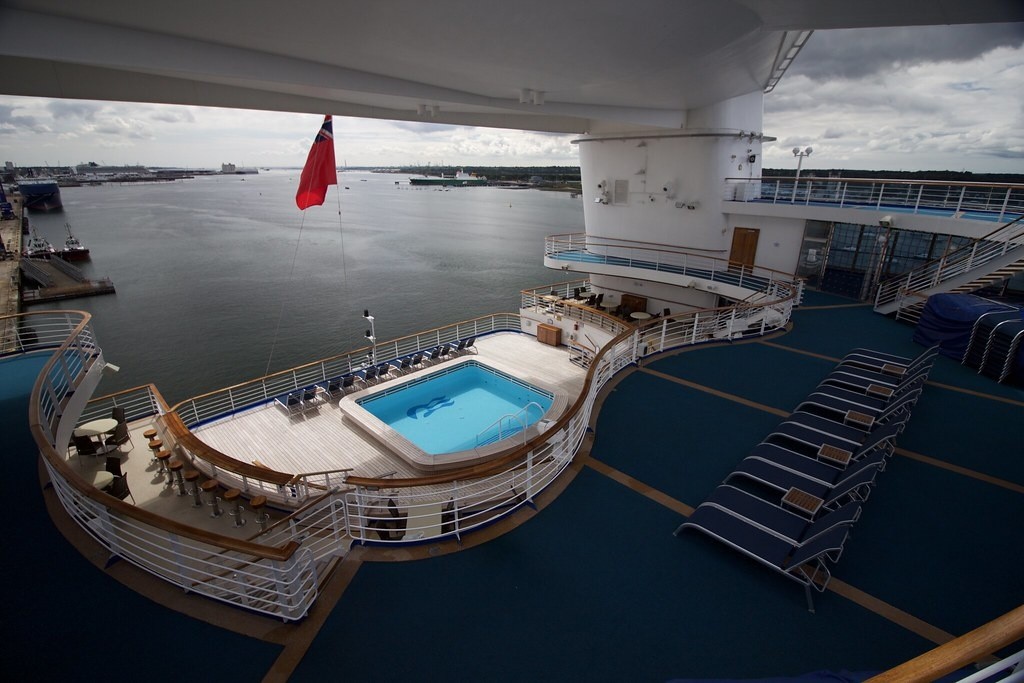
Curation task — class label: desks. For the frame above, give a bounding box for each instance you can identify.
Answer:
[630,311,651,335]
[671,312,692,337]
[543,295,561,313]
[81,471,113,490]
[600,302,618,314]
[579,292,595,303]
[405,503,443,540]
[74,419,118,456]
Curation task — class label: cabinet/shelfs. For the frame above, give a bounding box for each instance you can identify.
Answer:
[537,323,562,347]
[673,338,947,615]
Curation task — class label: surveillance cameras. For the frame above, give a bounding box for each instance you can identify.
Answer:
[598,180,609,197]
[663,182,673,198]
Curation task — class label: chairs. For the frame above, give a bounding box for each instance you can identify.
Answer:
[442,497,462,534]
[101,457,123,492]
[105,472,136,522]
[105,422,134,455]
[574,287,586,303]
[376,519,405,540]
[273,337,478,419]
[610,304,623,325]
[72,435,102,467]
[539,291,565,308]
[649,308,677,329]
[585,294,604,308]
[104,407,132,440]
[387,499,408,534]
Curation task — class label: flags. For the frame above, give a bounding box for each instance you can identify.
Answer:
[296,114,338,210]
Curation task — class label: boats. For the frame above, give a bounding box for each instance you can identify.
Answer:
[21,222,57,262]
[59,221,91,261]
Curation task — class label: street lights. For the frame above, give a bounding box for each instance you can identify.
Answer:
[789,146,815,204]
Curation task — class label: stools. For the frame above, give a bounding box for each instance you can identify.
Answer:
[143,429,269,534]
